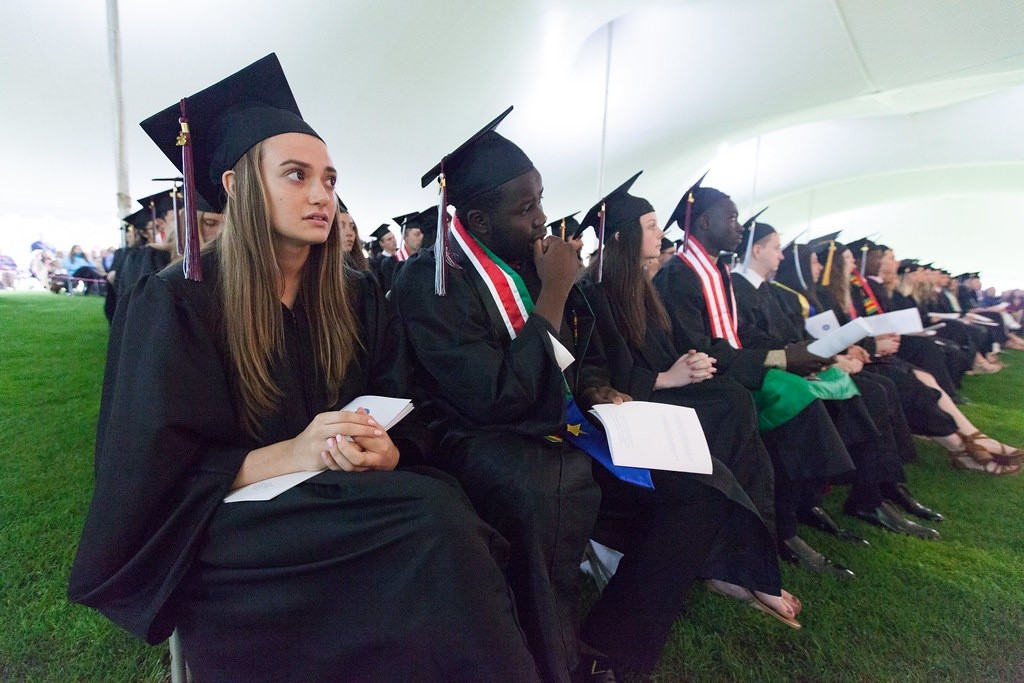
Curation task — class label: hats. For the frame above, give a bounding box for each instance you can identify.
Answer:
[785,230,981,290]
[545,211,580,243]
[336,193,438,266]
[127,178,185,256]
[661,171,731,253]
[574,171,656,284]
[422,106,533,296]
[138,52,327,280]
[740,206,776,273]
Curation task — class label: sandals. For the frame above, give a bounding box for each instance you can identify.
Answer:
[949,449,1020,475]
[962,429,1024,464]
[705,579,801,629]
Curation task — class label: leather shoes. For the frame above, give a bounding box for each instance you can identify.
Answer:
[778,536,855,578]
[805,507,870,549]
[845,500,940,541]
[889,490,943,521]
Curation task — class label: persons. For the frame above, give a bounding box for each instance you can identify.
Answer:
[646,232,676,276]
[103,177,225,324]
[548,210,584,253]
[578,170,756,599]
[728,209,1024,546]
[0,254,19,290]
[652,171,854,579]
[69,51,580,683]
[334,193,453,290]
[387,105,804,683]
[30,245,115,295]
